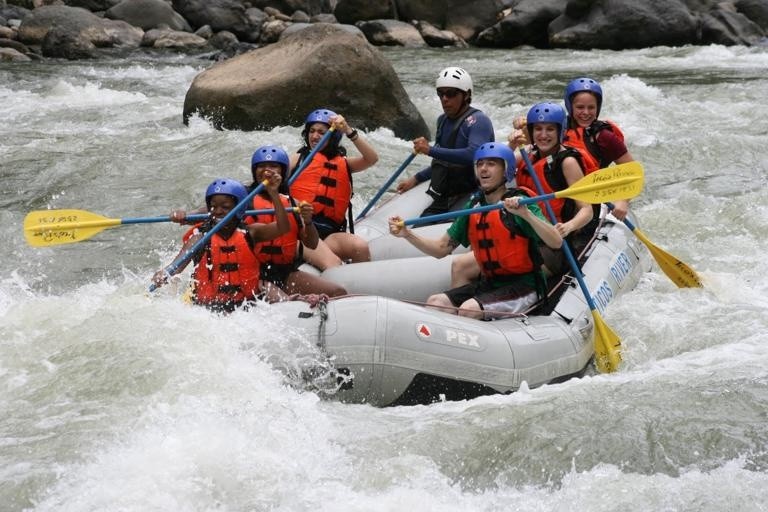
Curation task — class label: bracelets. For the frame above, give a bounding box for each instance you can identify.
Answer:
[304,220,313,227]
[351,134,359,142]
[346,127,357,139]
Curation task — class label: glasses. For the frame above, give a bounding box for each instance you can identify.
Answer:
[437,89,457,98]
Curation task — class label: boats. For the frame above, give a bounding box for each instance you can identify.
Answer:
[250,178,642,407]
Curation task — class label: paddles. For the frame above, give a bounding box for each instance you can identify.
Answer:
[23,206,300,246]
[603,202,704,289]
[394,161,644,226]
[519,143,624,374]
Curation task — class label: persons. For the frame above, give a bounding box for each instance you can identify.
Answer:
[387,142,564,321]
[450,99,594,288]
[169,142,347,305]
[395,65,495,229]
[288,108,380,272]
[511,77,636,222]
[151,167,291,316]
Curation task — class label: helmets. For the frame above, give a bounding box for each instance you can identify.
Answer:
[565,78,602,117]
[252,146,289,179]
[527,103,566,143]
[436,67,473,96]
[305,109,342,142]
[206,179,247,219]
[474,142,515,182]
